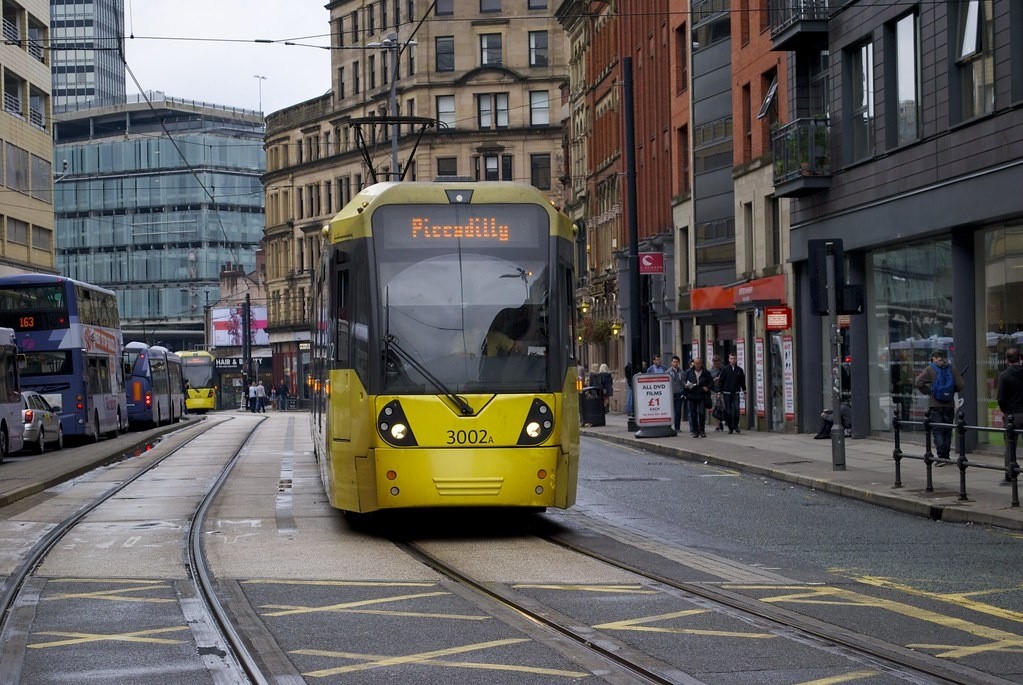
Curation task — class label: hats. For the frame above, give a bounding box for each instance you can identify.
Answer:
[930,350,944,362]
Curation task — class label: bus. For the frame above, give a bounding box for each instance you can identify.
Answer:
[310,116,579,515]
[174,350,218,415]
[123,341,189,428]
[0,274,130,442]
[0,327,26,465]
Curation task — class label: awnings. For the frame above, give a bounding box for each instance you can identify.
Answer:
[889,331,1023,350]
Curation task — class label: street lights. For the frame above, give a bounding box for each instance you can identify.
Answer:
[255,74,267,117]
[366,35,417,182]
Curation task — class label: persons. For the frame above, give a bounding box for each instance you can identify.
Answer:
[814,397,852,439]
[249,381,266,413]
[624,361,646,416]
[275,379,289,411]
[451,308,524,358]
[589,363,614,414]
[891,351,916,432]
[578,359,585,388]
[997,347,1023,485]
[646,353,747,438]
[915,349,965,467]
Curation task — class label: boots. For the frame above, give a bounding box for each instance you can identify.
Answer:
[604,404,610,414]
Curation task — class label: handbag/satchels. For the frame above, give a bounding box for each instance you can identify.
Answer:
[705,391,712,409]
[712,399,728,420]
[254,388,257,395]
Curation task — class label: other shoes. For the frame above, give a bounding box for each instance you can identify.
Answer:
[1005,473,1018,483]
[728,429,733,434]
[700,432,706,438]
[734,428,741,433]
[693,434,699,438]
[814,434,832,439]
[675,429,682,433]
[936,461,946,467]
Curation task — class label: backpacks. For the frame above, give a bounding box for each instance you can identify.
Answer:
[929,363,956,402]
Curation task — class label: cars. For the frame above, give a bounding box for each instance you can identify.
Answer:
[21,391,63,454]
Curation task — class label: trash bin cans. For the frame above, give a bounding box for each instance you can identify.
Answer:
[579,386,605,427]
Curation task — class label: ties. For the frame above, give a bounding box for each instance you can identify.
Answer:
[480,331,488,355]
